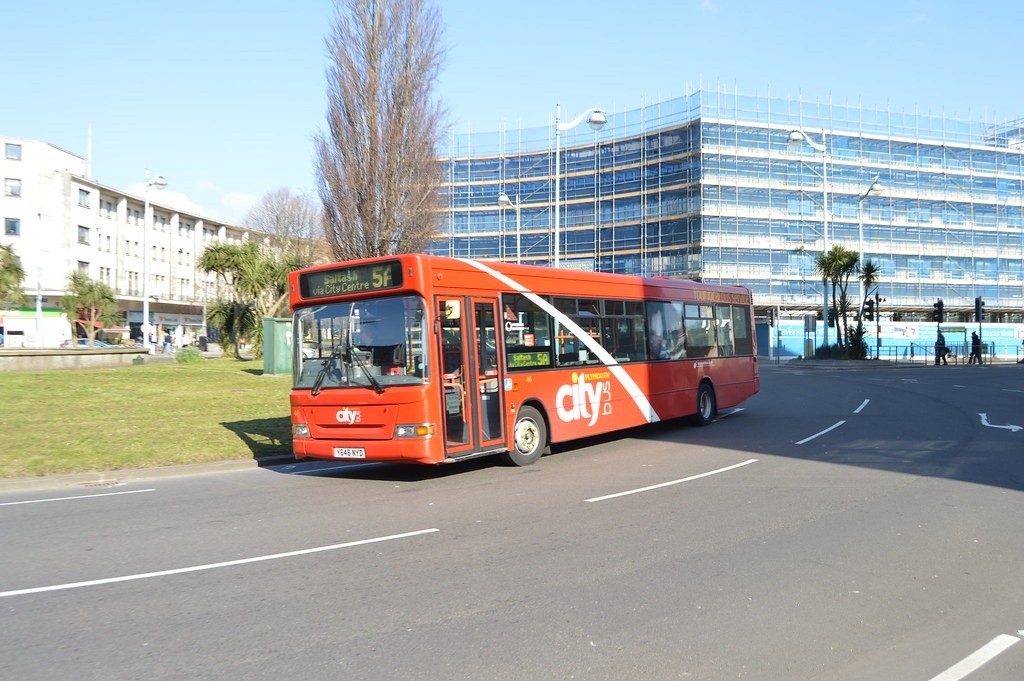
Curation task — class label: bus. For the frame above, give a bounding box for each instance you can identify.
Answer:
[288,253,761,466]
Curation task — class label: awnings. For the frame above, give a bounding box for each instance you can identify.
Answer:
[78,320,130,333]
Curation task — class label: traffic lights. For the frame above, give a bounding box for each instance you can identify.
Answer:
[864,299,874,321]
[933,300,943,323]
[975,297,986,322]
[828,309,834,327]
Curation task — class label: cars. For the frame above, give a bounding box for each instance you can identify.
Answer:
[63,338,113,348]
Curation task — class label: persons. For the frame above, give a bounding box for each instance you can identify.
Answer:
[360,326,393,375]
[967,332,983,364]
[934,330,948,365]
[650,335,668,359]
[161,330,176,354]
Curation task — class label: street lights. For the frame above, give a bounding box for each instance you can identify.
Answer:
[498,192,519,264]
[786,127,827,346]
[857,176,884,336]
[555,102,609,268]
[143,171,167,356]
